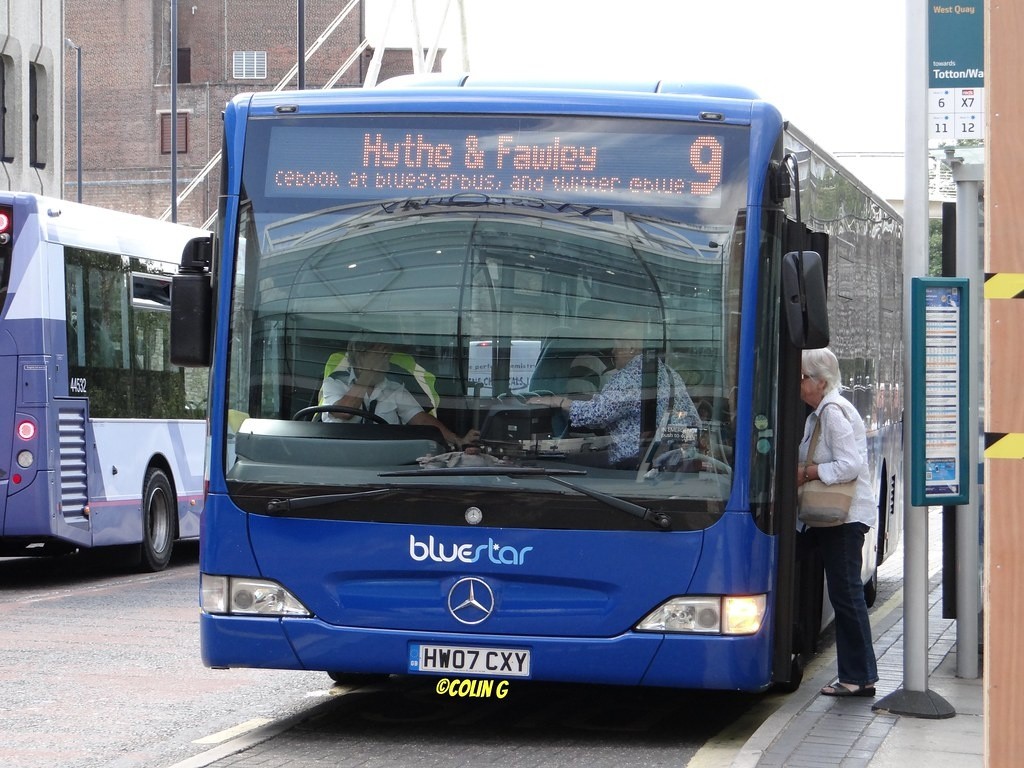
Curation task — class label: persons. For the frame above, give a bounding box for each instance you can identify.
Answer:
[797,348,880,697]
[323,328,480,451]
[526,316,702,471]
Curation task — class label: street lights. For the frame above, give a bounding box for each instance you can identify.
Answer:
[64,39,83,205]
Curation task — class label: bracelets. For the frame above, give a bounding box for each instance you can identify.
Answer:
[803,463,810,482]
[560,398,568,410]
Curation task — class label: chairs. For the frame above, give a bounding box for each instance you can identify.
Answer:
[308,327,737,424]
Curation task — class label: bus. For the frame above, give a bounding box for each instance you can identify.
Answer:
[0,193,302,574]
[167,70,904,694]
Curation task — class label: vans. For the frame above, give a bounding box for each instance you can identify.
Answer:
[466,341,545,396]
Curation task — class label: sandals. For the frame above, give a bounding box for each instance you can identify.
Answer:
[819,682,876,697]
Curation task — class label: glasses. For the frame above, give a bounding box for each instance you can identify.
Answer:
[801,373,817,380]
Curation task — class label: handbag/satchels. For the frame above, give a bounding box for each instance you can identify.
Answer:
[797,402,857,527]
